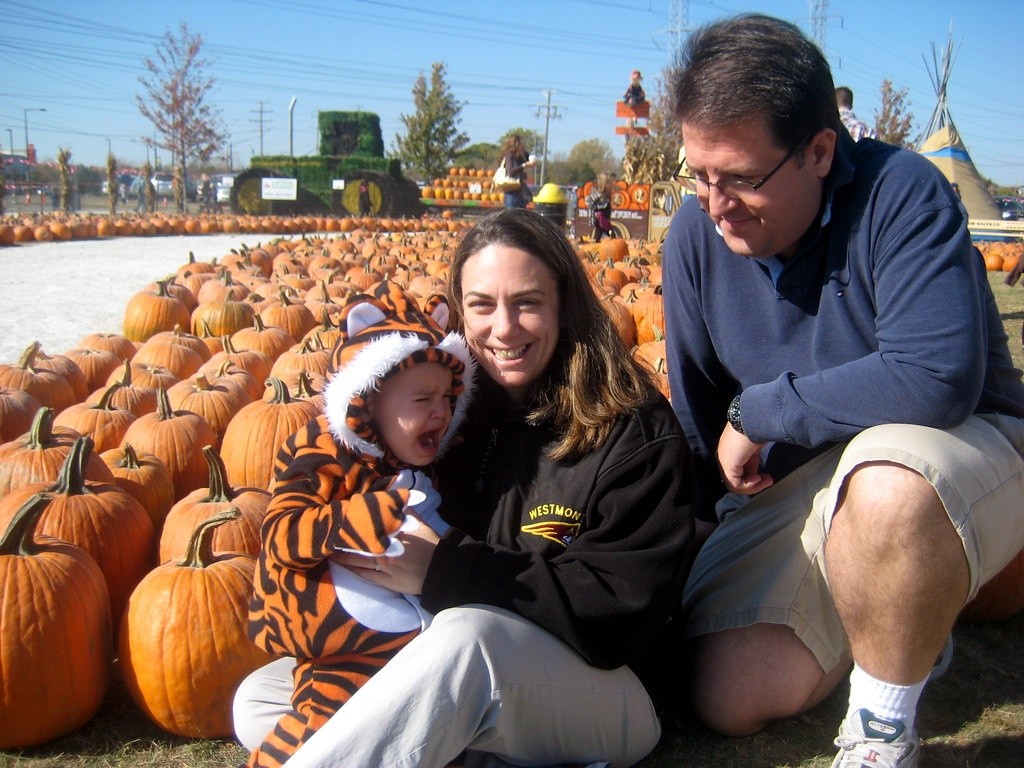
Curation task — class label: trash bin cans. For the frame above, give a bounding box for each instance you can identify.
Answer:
[532,183,569,229]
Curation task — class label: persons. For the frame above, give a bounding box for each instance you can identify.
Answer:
[198,173,224,214]
[117,169,147,212]
[232,209,718,768]
[358,178,375,218]
[586,172,623,244]
[50,186,60,210]
[662,12,1024,768]
[497,133,538,210]
[623,70,645,126]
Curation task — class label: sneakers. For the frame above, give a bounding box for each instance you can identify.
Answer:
[830,707,919,768]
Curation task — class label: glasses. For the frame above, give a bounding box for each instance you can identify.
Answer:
[672,130,812,199]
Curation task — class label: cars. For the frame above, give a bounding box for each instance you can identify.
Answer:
[993,195,1024,221]
[101,169,238,206]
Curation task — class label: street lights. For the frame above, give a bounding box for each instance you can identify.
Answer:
[105,137,112,159]
[4,128,17,184]
[24,107,47,194]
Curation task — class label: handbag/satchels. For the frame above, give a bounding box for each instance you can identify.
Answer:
[489,156,520,191]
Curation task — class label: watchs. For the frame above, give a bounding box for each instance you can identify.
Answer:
[727,395,744,436]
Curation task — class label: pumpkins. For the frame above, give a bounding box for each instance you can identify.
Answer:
[0,168,669,749]
[971,239,1023,272]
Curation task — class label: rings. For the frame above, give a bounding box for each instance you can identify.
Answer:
[375,559,381,571]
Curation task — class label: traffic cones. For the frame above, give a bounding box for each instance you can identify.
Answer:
[22,192,32,206]
